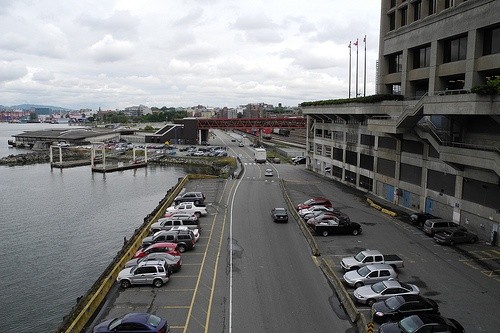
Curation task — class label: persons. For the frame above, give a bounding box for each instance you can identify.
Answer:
[231,171,238,179]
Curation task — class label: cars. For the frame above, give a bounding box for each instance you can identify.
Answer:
[239,143,244,146]
[92,312,170,333]
[274,158,281,164]
[353,280,421,308]
[291,156,311,165]
[271,207,289,223]
[370,294,441,324]
[377,312,466,333]
[264,169,274,176]
[231,138,236,142]
[434,228,478,246]
[249,143,258,148]
[97,142,228,157]
[296,198,351,226]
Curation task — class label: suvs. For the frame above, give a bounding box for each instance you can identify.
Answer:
[343,264,400,288]
[409,213,442,226]
[116,192,208,288]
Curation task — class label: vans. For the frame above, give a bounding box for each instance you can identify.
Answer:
[423,219,467,236]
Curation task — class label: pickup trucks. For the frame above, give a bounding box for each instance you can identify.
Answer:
[314,217,363,237]
[340,249,405,273]
[57,142,71,147]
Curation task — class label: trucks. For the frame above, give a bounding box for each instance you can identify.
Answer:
[254,147,267,163]
